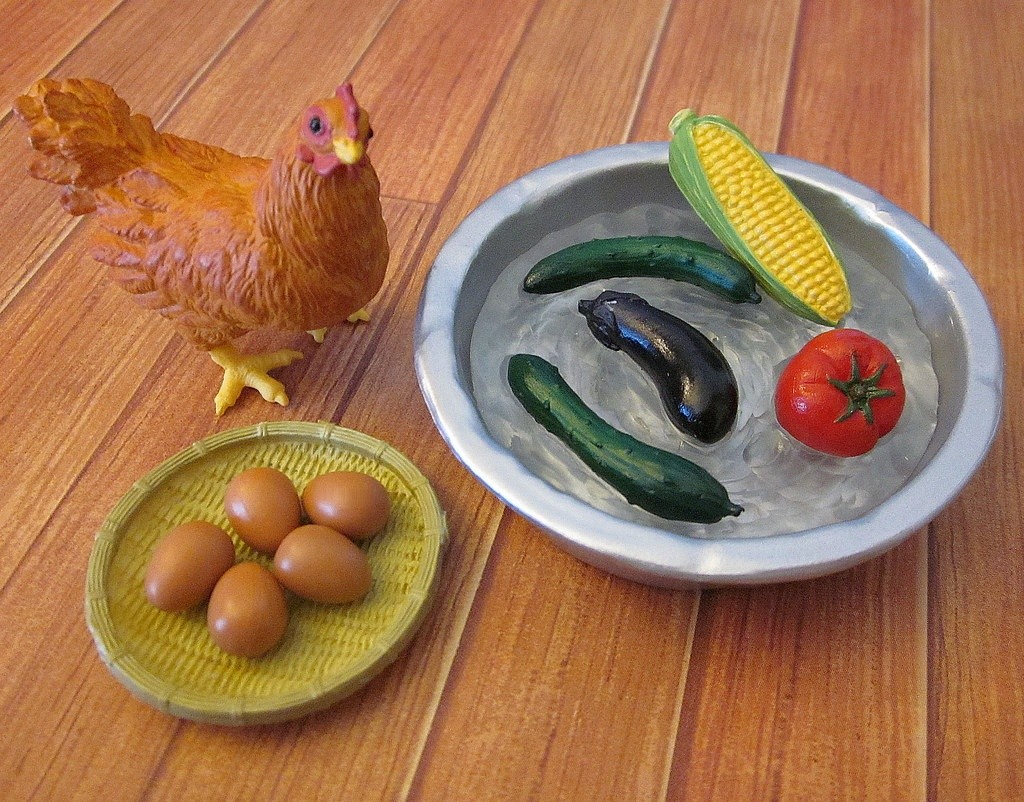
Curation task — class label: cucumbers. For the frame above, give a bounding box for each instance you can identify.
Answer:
[522,237,763,306]
[507,352,744,523]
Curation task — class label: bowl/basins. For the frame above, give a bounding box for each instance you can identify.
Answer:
[409,141,1004,593]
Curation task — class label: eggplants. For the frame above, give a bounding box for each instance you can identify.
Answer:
[576,291,738,443]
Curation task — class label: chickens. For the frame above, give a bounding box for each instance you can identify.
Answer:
[10,75,390,417]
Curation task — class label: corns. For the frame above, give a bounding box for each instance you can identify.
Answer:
[666,108,852,330]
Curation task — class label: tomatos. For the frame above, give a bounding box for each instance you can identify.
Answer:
[774,328,904,456]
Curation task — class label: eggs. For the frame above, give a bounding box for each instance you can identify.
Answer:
[143,467,391,661]
